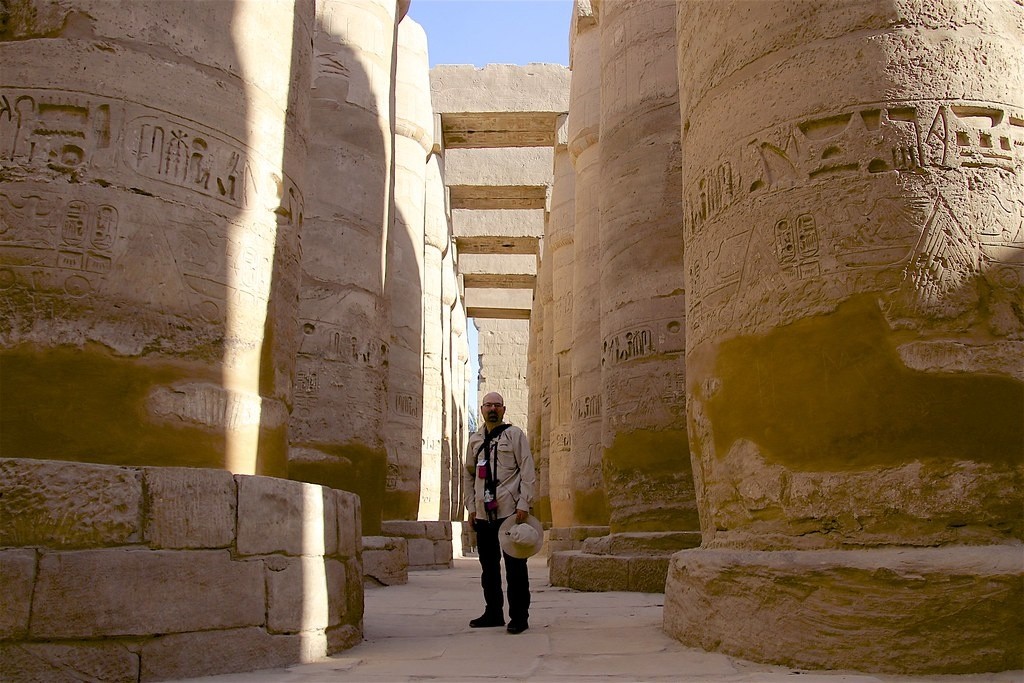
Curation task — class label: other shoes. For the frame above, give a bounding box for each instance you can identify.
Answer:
[508,617,528,630]
[469,610,505,627]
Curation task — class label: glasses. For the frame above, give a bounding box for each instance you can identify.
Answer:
[483,403,504,408]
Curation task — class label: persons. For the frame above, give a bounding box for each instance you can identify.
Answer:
[463,392,536,634]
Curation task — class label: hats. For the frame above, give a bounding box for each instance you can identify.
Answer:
[499,513,544,559]
